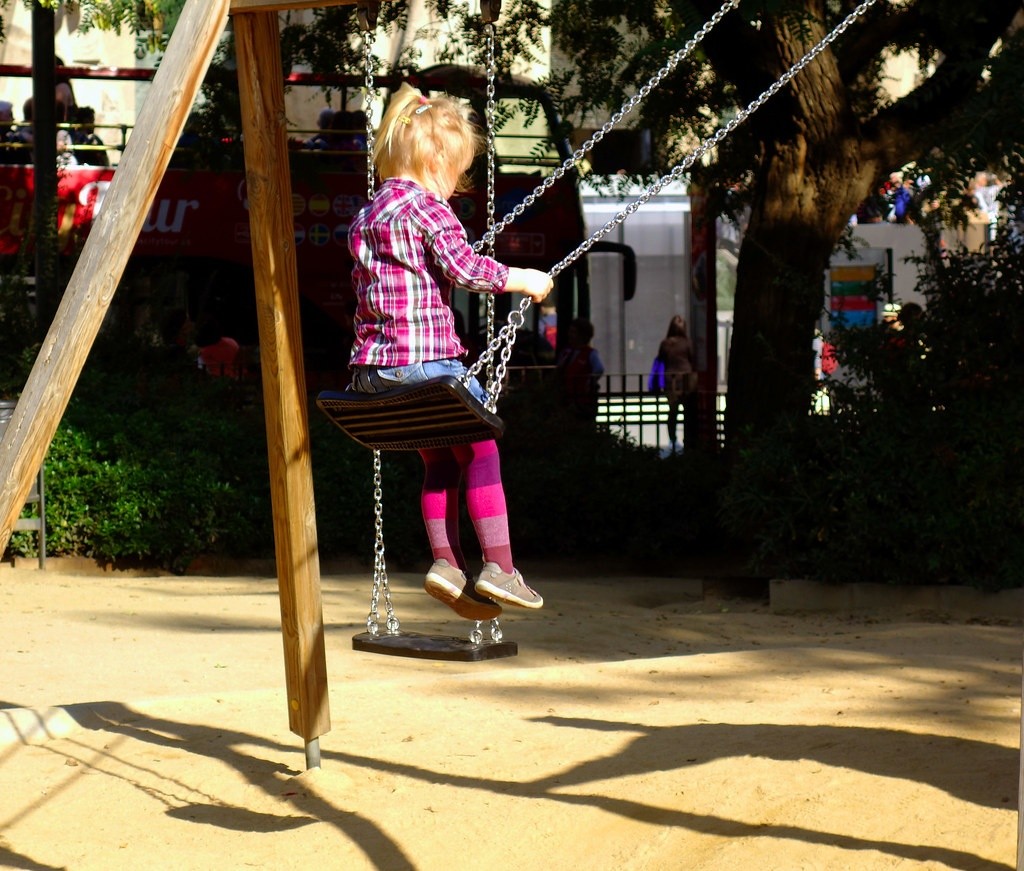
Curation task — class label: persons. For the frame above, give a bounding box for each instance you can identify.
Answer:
[178,109,369,172]
[880,304,924,362]
[821,325,847,381]
[559,318,603,430]
[152,312,199,368]
[348,81,552,618]
[7,99,35,143]
[811,329,830,415]
[193,316,248,379]
[649,316,691,449]
[0,106,31,165]
[73,107,107,164]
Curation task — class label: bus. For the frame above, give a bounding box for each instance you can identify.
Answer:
[0,65,639,405]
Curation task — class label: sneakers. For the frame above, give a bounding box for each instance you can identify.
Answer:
[474,562,543,608]
[424,560,502,621]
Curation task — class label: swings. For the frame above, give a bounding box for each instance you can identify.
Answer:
[353,26,517,663]
[319,1,876,449]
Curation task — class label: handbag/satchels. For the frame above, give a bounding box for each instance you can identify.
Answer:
[648,343,667,394]
